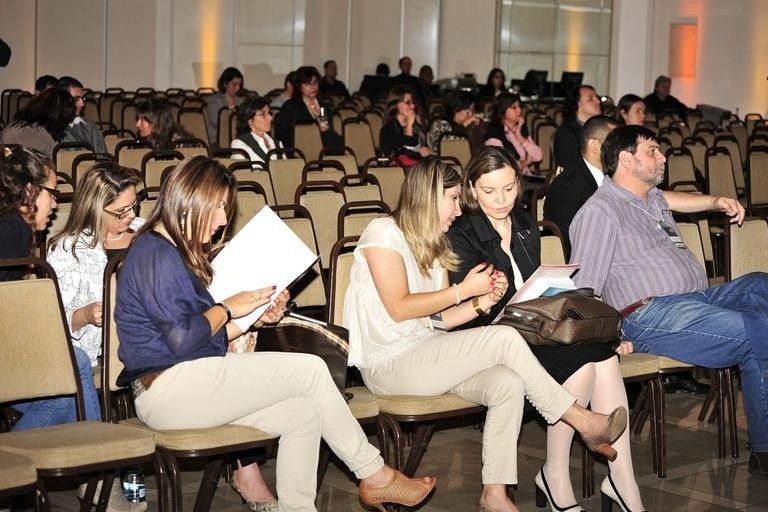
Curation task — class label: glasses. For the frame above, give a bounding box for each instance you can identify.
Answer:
[463,108,475,115]
[509,104,522,110]
[103,196,141,219]
[75,95,88,103]
[584,137,606,145]
[254,111,271,118]
[38,185,60,200]
[402,100,415,106]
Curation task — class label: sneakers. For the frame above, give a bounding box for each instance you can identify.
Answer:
[748,452,767,476]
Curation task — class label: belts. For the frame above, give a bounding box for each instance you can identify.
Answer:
[129,371,164,398]
[620,298,655,321]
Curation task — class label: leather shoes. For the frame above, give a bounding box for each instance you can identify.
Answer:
[664,372,711,394]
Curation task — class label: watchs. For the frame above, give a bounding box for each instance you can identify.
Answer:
[472,295,491,318]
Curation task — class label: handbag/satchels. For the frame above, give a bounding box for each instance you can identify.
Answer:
[254,313,354,405]
[497,287,624,347]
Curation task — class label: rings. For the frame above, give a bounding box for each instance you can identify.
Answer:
[254,295,261,300]
[498,290,503,298]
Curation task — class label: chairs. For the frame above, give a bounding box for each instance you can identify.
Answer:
[554,72,584,97]
[519,70,547,97]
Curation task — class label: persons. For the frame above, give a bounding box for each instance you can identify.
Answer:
[55,76,108,154]
[614,94,647,128]
[552,85,602,170]
[361,64,391,98]
[274,66,345,159]
[394,57,420,90]
[37,75,58,97]
[379,84,430,165]
[45,161,279,512]
[115,155,438,512]
[1,88,77,159]
[418,66,440,94]
[133,99,208,162]
[444,144,646,512]
[207,68,249,153]
[425,89,484,157]
[341,155,628,512]
[567,124,768,477]
[542,114,623,264]
[479,67,509,98]
[0,143,103,433]
[483,92,544,177]
[266,70,296,108]
[319,61,350,100]
[642,75,689,122]
[230,96,288,172]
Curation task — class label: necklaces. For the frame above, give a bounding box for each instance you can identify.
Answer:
[310,102,317,109]
[104,232,124,242]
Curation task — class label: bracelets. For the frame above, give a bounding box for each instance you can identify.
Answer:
[452,282,461,306]
[212,302,233,323]
[712,195,722,210]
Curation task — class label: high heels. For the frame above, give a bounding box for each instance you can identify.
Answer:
[477,501,498,512]
[77,471,148,512]
[583,406,627,462]
[359,469,437,512]
[230,470,277,512]
[534,466,586,512]
[600,474,646,512]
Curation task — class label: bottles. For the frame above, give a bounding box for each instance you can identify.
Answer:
[121,470,146,503]
